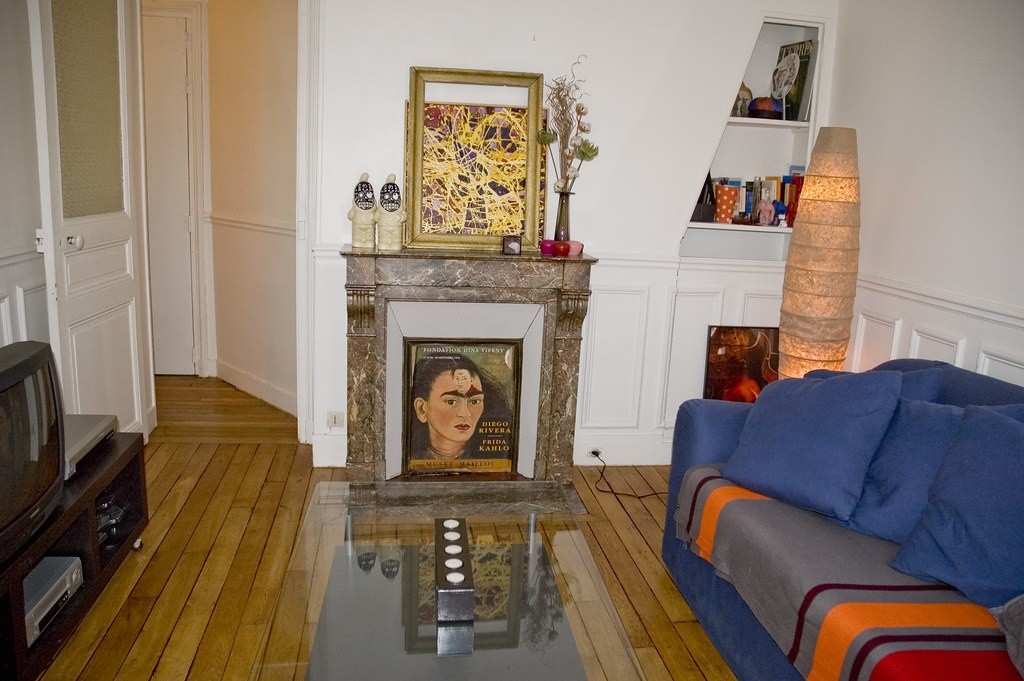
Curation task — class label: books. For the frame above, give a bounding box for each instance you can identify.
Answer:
[769,38,819,121]
[712,162,805,228]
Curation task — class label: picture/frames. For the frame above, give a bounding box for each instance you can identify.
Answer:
[403,66,543,250]
[402,337,524,473]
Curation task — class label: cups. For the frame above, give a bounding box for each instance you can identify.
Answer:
[714,184,739,224]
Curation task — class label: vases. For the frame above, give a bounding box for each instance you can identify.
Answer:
[553,191,576,241]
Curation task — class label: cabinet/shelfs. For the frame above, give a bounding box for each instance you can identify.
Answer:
[0,432,150,681]
[688,18,825,234]
[255,481,646,681]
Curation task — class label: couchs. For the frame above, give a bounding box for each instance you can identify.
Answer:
[663,359,1024,681]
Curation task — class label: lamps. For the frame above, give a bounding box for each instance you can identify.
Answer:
[777,126,860,382]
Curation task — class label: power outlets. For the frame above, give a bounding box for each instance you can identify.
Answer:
[588,446,602,459]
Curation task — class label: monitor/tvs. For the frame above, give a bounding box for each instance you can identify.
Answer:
[0,341,66,565]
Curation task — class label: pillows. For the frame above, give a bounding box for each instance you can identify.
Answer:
[723,370,901,520]
[803,366,941,403]
[826,397,964,545]
[887,404,1024,609]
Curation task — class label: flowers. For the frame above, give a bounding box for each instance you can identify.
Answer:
[536,53,598,240]
